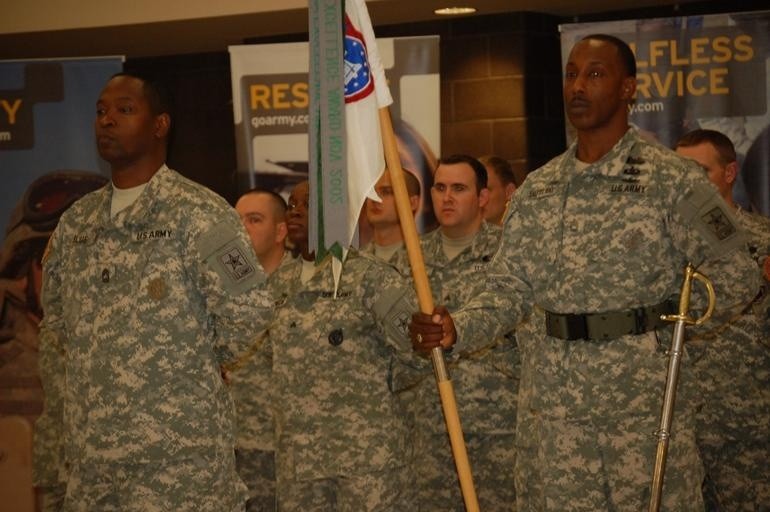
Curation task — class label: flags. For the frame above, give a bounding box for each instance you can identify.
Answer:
[308,0,395,301]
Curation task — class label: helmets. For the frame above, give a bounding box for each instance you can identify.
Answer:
[0,169,109,279]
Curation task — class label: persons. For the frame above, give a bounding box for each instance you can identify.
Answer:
[0,171,110,512]
[214,156,521,510]
[409,34,762,512]
[671,129,770,512]
[31,72,280,512]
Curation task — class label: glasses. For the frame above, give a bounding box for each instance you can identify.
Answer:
[23,173,109,230]
